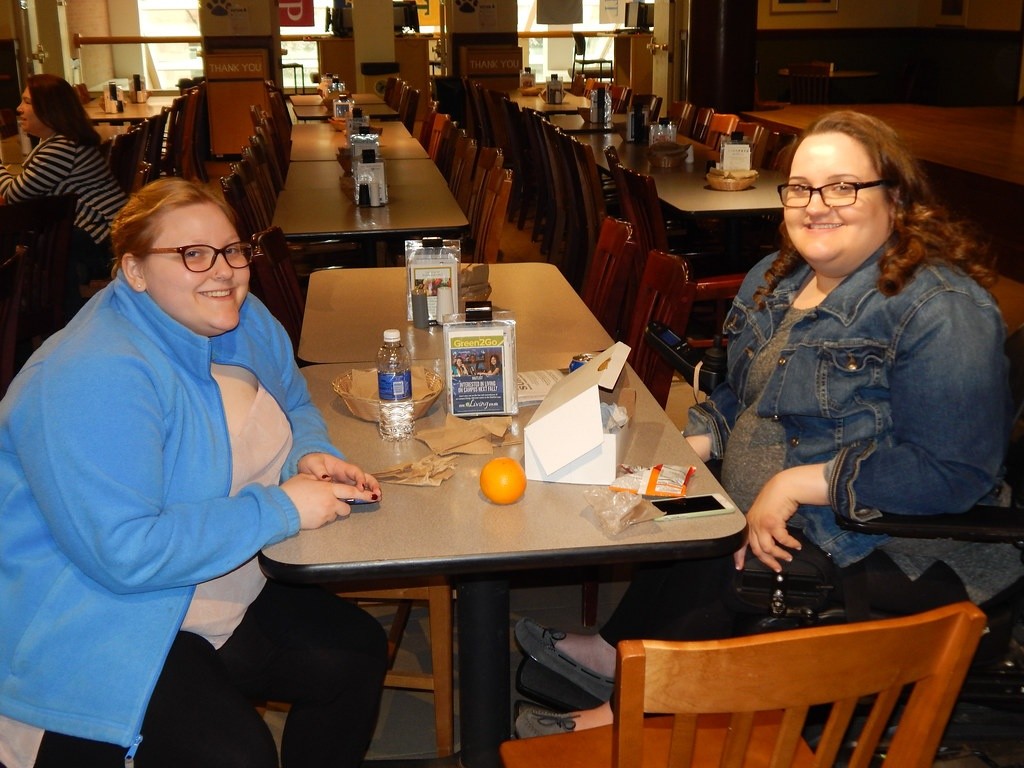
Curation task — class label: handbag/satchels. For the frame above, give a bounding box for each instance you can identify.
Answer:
[726,556,846,636]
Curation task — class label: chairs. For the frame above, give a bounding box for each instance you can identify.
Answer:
[409,73,753,349]
[579,249,695,628]
[220,62,360,365]
[499,600,987,768]
[569,31,800,171]
[384,73,513,263]
[580,215,638,337]
[802,502,1023,767]
[0,80,209,379]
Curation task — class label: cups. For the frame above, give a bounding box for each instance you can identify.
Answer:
[438,286,453,325]
[360,183,370,208]
[370,182,381,206]
[138,91,146,102]
[412,289,428,328]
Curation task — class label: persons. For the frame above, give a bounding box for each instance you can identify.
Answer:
[0,176,382,768]
[515,110,1012,738]
[451,354,501,376]
[0,74,130,379]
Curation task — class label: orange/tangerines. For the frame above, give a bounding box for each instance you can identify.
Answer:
[480,457,526,505]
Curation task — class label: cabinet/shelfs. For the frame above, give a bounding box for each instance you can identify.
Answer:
[301,35,439,120]
[614,34,653,96]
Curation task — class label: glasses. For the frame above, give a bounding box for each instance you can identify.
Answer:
[146,241,257,273]
[777,179,882,208]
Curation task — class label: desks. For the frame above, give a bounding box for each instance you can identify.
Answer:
[270,94,470,268]
[733,104,1024,195]
[259,351,750,768]
[299,260,613,363]
[508,91,789,215]
[0,96,180,168]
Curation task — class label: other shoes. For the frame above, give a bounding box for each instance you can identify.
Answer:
[515,708,580,740]
[516,615,614,702]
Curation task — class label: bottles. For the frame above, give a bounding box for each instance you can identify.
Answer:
[378,329,415,442]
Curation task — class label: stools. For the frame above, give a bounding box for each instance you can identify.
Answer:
[256,583,453,759]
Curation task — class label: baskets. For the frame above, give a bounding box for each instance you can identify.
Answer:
[706,172,759,191]
[329,367,446,422]
[540,92,566,101]
[127,91,153,104]
[318,88,384,136]
[335,146,352,172]
[577,107,590,121]
[644,152,688,167]
[341,182,390,200]
[517,87,544,96]
[99,101,127,111]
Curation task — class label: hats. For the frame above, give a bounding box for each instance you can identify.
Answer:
[176,79,193,88]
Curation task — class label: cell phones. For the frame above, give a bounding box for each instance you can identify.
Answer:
[650,494,736,522]
